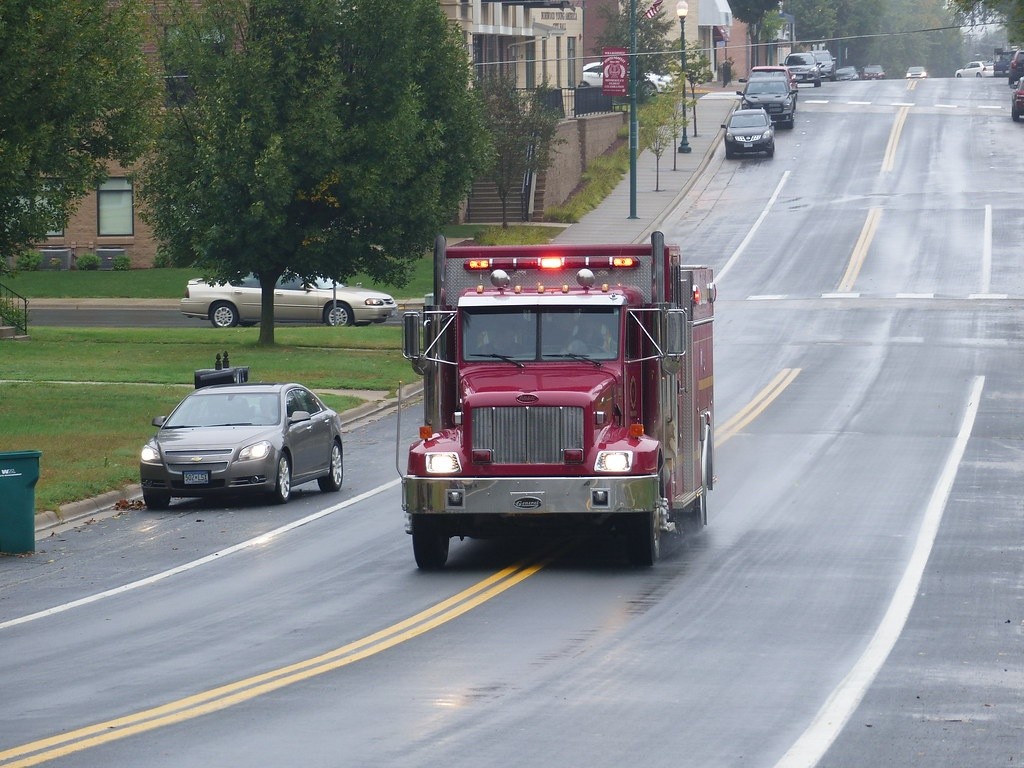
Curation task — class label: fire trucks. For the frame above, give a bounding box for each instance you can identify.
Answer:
[396,232,715,573]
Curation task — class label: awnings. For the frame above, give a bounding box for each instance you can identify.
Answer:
[712,25,732,43]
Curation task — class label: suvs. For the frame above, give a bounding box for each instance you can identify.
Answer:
[1008,49,1024,85]
[779,51,822,87]
[1010,77,1024,121]
[805,50,837,82]
[738,65,798,99]
[860,64,886,81]
[993,49,1016,76]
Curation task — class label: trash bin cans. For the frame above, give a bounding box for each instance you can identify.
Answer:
[0,449,41,555]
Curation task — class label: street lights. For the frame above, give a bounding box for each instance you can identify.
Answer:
[676,1,692,154]
[817,35,825,49]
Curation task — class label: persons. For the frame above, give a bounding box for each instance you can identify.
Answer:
[476,320,529,359]
[557,312,605,354]
[721,57,735,87]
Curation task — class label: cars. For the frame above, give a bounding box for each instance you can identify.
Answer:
[736,78,799,129]
[139,382,344,512]
[179,271,398,328]
[833,65,861,81]
[904,66,929,80]
[721,109,777,158]
[583,63,676,97]
[954,59,994,78]
